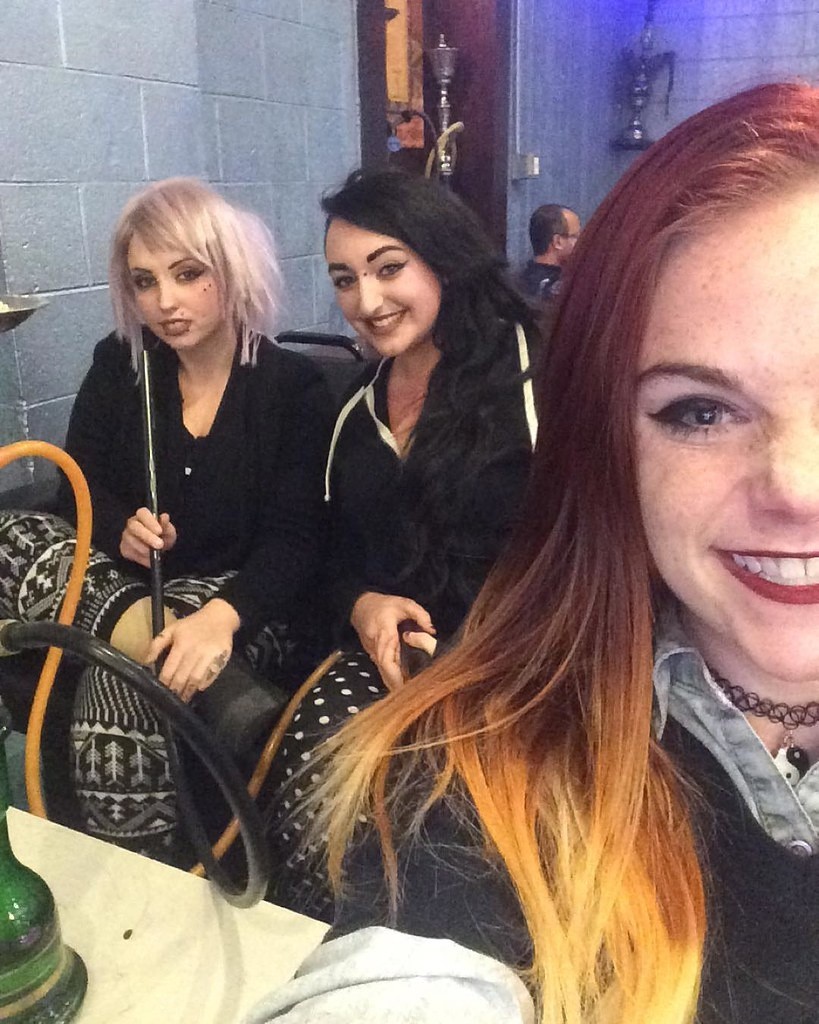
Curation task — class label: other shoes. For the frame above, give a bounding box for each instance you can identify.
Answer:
[191,653,286,753]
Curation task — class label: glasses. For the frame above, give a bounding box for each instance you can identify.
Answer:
[552,228,582,239]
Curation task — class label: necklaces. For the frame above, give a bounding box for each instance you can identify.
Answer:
[704,661,819,787]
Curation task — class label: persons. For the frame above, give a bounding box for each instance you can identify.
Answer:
[270,163,550,924]
[519,204,582,298]
[238,79,819,1024]
[0,177,324,863]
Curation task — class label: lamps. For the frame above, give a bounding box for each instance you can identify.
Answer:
[608,0,676,152]
[423,33,465,187]
